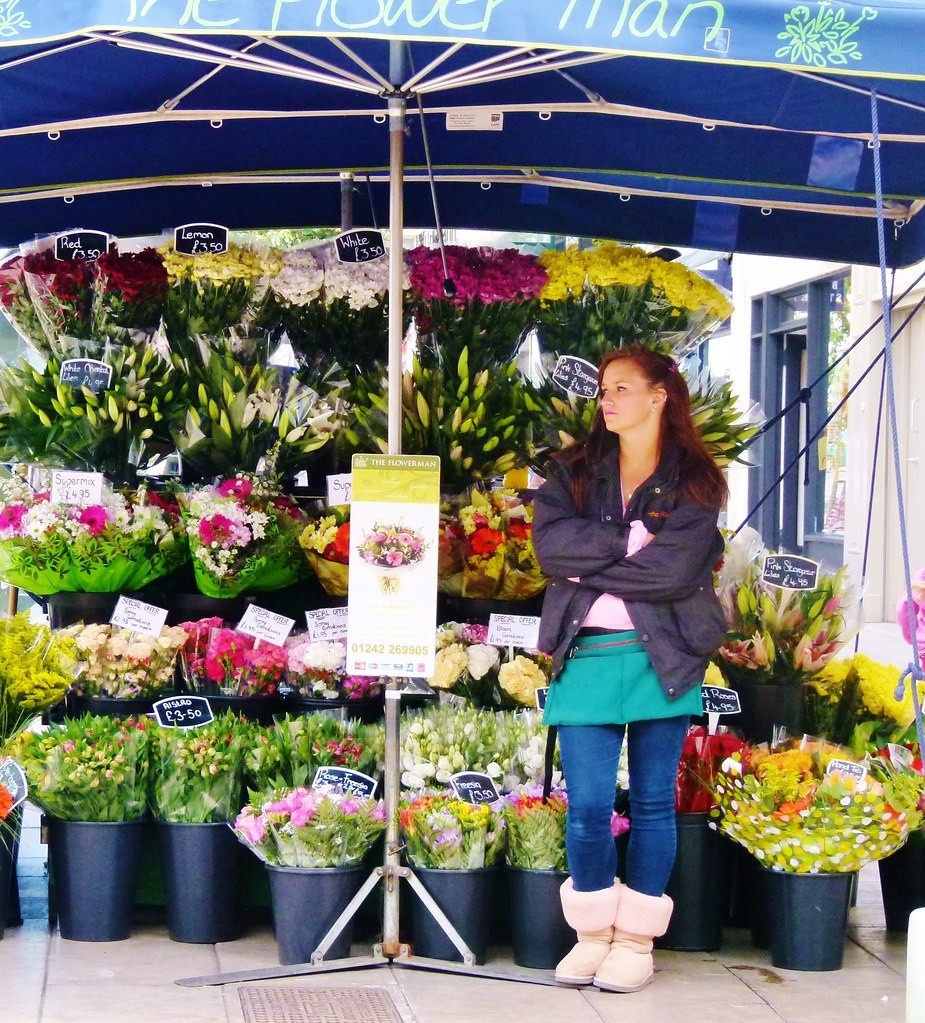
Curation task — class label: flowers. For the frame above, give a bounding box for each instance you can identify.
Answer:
[0,242,925,875]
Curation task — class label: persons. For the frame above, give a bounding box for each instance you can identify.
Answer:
[532,344,724,993]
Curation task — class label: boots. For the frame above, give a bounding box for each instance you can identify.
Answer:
[593,883,673,992]
[554,876,621,984]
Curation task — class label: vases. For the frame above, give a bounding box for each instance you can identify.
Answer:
[288,695,358,720]
[182,458,215,485]
[739,684,808,745]
[762,869,860,972]
[193,694,259,721]
[408,866,488,965]
[0,821,16,939]
[164,590,246,628]
[505,868,579,970]
[306,452,351,486]
[48,818,132,943]
[81,695,152,714]
[653,811,724,951]
[441,594,535,628]
[54,592,142,628]
[266,866,360,965]
[160,820,235,943]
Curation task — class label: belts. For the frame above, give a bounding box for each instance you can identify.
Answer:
[574,627,625,637]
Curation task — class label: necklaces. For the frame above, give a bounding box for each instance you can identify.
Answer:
[623,469,655,499]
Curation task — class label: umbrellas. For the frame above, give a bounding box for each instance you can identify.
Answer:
[0,0,924,959]
